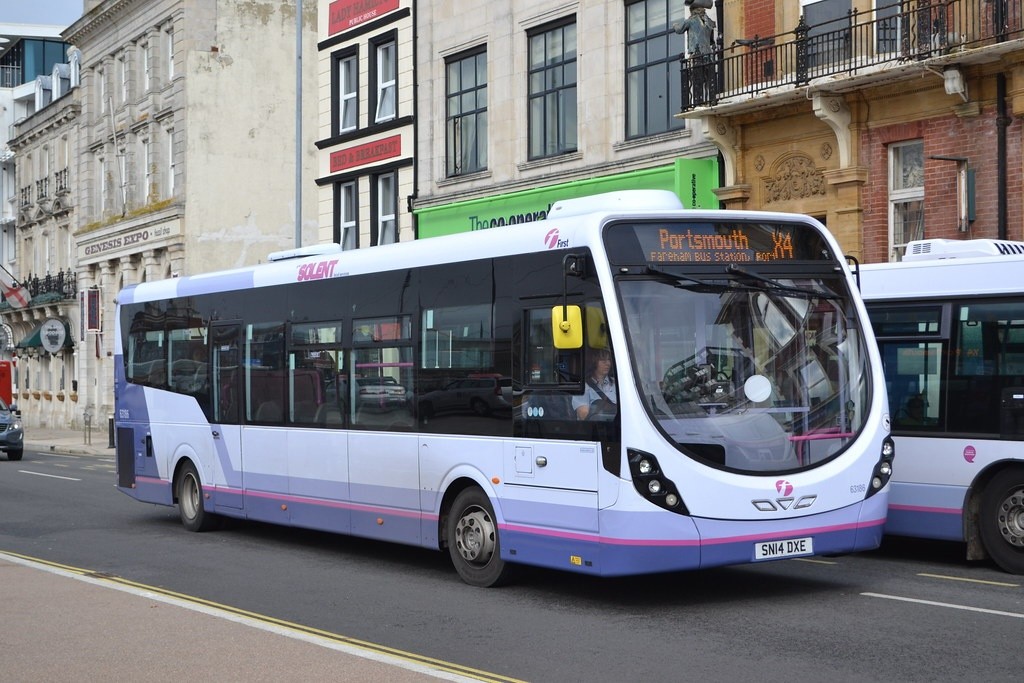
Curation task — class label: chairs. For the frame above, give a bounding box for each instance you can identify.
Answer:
[147,356,416,429]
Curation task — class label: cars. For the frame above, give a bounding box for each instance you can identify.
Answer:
[323,374,406,411]
[0,398,24,461]
[407,374,534,422]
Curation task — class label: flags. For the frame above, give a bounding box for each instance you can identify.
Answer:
[0,265,32,309]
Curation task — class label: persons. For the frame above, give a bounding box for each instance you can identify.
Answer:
[571,347,618,420]
[672,4,718,108]
[187,346,209,375]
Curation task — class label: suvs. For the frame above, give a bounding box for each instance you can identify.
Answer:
[261,343,336,382]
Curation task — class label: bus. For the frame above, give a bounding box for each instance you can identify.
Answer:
[113,189,895,588]
[623,239,1024,575]
